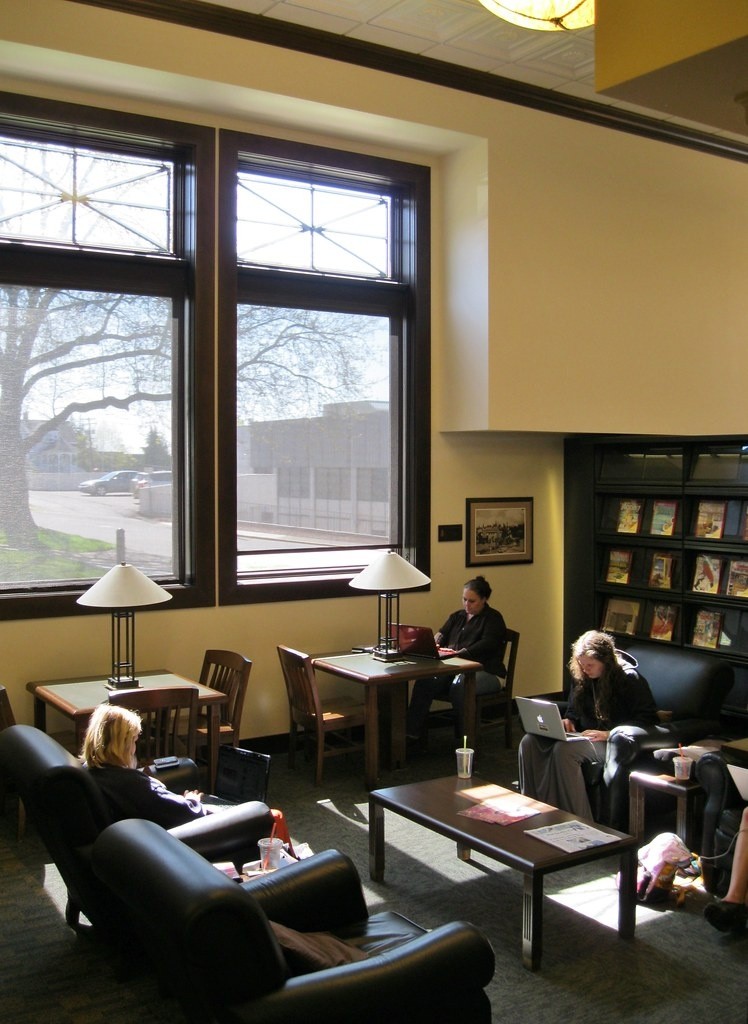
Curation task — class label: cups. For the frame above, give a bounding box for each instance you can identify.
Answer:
[672,757,692,785]
[455,748,474,779]
[257,837,283,874]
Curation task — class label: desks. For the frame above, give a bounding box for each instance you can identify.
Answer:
[307,644,485,775]
[35,671,228,795]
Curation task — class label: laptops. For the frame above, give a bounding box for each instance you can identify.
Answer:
[388,623,459,659]
[514,696,596,741]
[726,764,748,801]
[200,744,272,813]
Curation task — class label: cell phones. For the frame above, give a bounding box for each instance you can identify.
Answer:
[153,756,179,765]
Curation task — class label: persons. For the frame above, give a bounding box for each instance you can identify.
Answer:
[405,575,508,756]
[704,808,748,940]
[77,706,300,861]
[518,630,654,820]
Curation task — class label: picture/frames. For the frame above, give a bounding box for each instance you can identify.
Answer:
[467,496,536,568]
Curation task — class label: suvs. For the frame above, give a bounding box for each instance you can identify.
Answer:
[131,472,173,498]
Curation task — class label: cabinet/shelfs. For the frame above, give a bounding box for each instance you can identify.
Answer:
[563,433,748,727]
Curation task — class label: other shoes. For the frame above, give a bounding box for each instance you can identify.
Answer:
[704,901,748,933]
[405,728,420,740]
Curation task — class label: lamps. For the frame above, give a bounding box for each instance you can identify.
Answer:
[347,550,431,664]
[75,562,176,690]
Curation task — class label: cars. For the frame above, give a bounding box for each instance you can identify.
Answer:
[77,469,139,497]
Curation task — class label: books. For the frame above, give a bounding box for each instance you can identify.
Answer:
[692,556,723,595]
[650,604,678,642]
[726,560,748,597]
[695,501,727,538]
[607,551,631,584]
[743,506,748,540]
[649,553,672,589]
[651,501,678,535]
[693,610,722,648]
[603,598,640,633]
[617,499,644,533]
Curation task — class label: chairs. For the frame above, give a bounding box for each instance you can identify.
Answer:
[1,631,748,1024]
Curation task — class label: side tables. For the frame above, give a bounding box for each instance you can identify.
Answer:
[629,768,702,865]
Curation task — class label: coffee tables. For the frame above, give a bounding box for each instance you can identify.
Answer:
[362,775,642,966]
